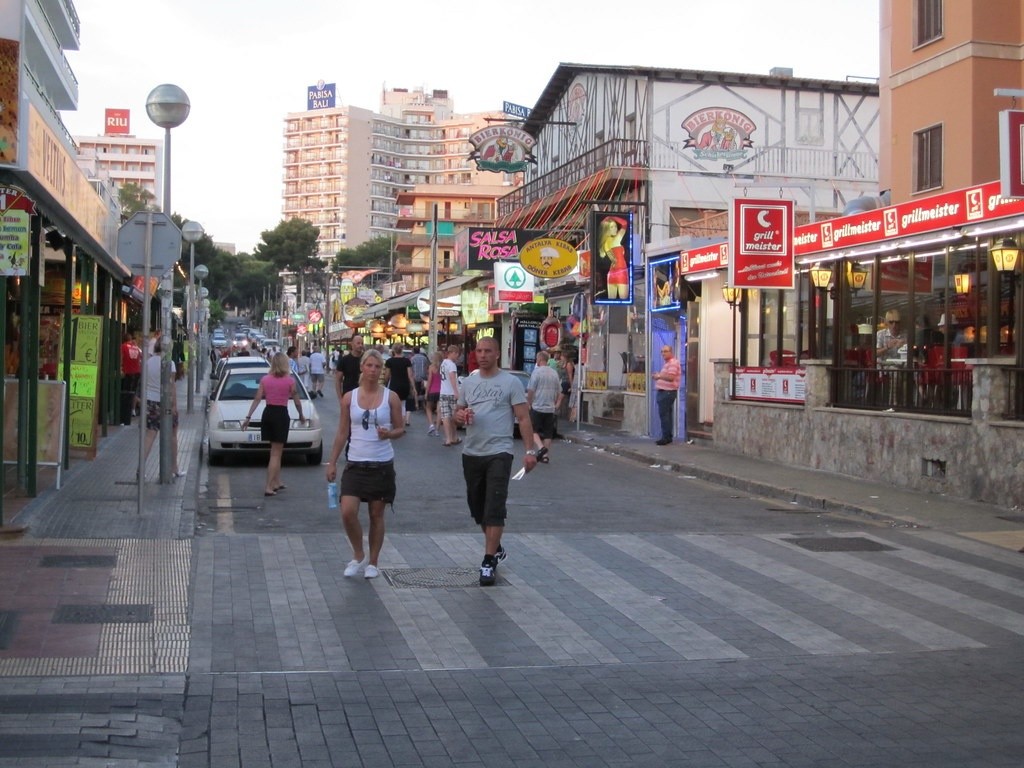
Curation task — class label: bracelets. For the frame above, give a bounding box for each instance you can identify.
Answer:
[245,416,251,419]
[658,373,660,379]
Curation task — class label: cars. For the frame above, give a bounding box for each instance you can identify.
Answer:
[211,355,272,394]
[212,319,281,360]
[206,367,323,468]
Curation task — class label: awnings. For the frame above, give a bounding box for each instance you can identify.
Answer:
[362,275,476,319]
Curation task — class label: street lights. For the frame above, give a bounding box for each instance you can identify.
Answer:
[145,83,179,488]
[198,286,210,380]
[194,263,210,393]
[182,220,206,415]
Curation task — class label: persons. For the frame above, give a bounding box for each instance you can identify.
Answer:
[598,216,628,299]
[327,348,405,578]
[119,330,187,479]
[452,335,537,586]
[526,351,563,464]
[877,310,966,409]
[207,339,278,374]
[652,345,682,445]
[329,346,391,383]
[548,349,576,393]
[241,353,306,496]
[334,335,364,461]
[384,341,462,447]
[287,344,325,399]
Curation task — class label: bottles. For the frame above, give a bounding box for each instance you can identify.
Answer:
[328,482,337,509]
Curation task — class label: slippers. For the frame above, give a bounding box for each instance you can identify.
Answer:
[264,491,279,496]
[452,437,463,444]
[441,441,451,446]
[274,484,288,491]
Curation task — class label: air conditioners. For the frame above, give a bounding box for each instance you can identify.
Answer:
[415,221,424,227]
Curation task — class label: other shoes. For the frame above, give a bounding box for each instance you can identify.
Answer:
[655,437,673,446]
[427,425,435,435]
[172,470,187,478]
[434,431,440,436]
[405,422,410,426]
[317,389,323,397]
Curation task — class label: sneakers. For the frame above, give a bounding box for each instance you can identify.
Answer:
[479,556,496,585]
[364,565,380,577]
[495,547,508,565]
[344,554,366,577]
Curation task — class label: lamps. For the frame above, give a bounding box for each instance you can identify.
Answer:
[809,261,839,300]
[952,257,976,296]
[846,260,869,298]
[720,281,743,313]
[987,237,1024,281]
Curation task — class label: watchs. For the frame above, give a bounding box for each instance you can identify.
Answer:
[525,450,536,456]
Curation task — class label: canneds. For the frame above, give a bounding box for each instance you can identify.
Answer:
[463,408,472,424]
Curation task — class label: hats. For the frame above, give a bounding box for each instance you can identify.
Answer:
[937,313,958,326]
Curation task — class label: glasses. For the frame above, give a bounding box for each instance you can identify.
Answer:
[362,410,369,430]
[887,320,899,324]
[661,350,670,353]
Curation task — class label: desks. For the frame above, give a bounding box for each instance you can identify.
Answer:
[782,354,810,359]
[885,358,919,407]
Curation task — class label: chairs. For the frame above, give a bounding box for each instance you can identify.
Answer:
[228,383,249,396]
[769,350,796,368]
[862,343,894,406]
[917,345,973,412]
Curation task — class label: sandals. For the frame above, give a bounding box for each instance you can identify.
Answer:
[541,457,549,463]
[537,447,548,461]
